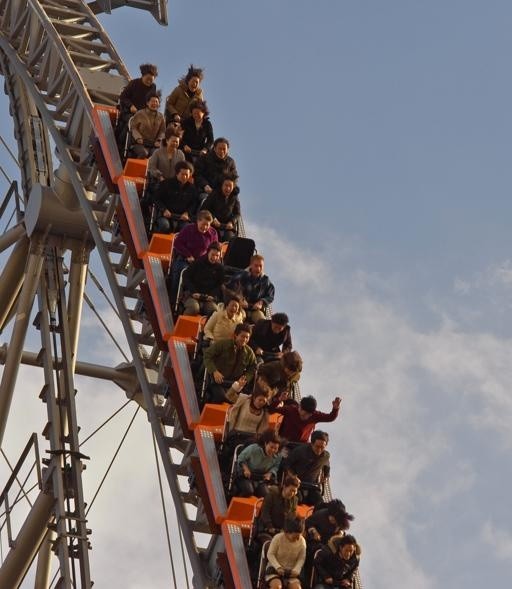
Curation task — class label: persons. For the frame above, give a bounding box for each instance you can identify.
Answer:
[204,296,362,589]
[120,64,275,322]
